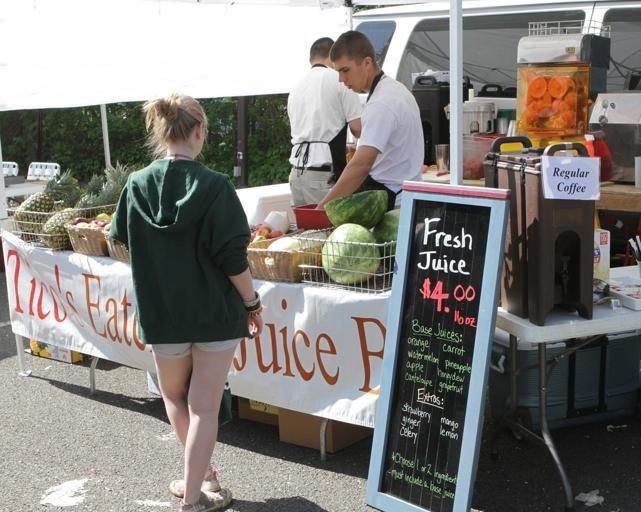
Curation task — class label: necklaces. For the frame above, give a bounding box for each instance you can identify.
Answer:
[161,148,193,163]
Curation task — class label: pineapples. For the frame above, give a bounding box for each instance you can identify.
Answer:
[13,159,144,248]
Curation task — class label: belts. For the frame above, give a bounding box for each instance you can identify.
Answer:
[294,166,332,173]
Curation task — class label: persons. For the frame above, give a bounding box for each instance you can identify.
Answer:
[315,29,426,222]
[104,93,264,512]
[285,36,366,212]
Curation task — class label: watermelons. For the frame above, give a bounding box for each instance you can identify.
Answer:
[321,189,400,284]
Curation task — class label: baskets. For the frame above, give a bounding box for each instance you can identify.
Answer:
[246,234,325,283]
[296,223,395,296]
[527,19,612,37]
[4,198,130,264]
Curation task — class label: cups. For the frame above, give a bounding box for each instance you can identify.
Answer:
[435,143,450,174]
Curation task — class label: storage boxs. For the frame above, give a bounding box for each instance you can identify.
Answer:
[236,387,373,454]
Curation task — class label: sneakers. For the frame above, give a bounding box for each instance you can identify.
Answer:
[167,474,221,498]
[179,485,234,512]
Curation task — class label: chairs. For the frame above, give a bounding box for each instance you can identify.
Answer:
[2,161,60,182]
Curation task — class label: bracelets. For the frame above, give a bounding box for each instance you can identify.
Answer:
[327,185,341,200]
[243,291,263,318]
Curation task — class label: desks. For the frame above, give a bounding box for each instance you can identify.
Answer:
[3,232,395,460]
[1,180,53,217]
[492,264,641,511]
